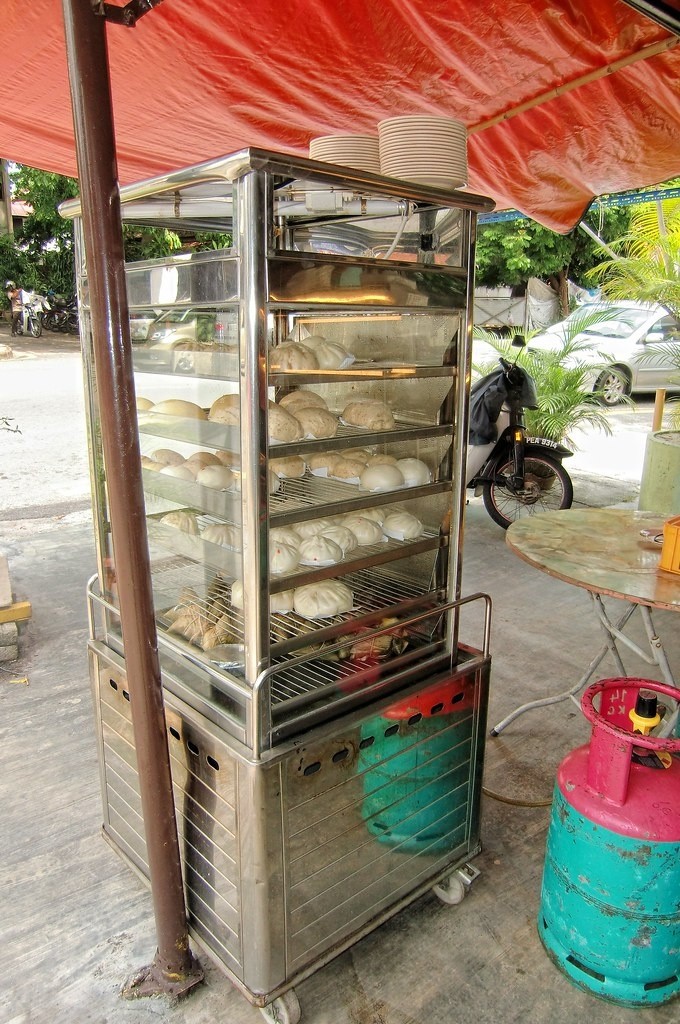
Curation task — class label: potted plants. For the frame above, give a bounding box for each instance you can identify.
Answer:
[474,307,638,490]
[585,177,680,514]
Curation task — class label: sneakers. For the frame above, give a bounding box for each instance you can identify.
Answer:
[11,331,17,336]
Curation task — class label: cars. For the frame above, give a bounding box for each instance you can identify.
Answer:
[128,309,163,344]
[530,299,680,408]
[144,305,293,375]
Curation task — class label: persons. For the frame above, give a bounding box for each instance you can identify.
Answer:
[5,280,23,337]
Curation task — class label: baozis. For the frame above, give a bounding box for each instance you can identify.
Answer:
[159,508,425,574]
[164,334,353,371]
[229,577,297,613]
[292,578,353,619]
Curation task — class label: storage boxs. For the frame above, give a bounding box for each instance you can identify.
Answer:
[657,515,680,575]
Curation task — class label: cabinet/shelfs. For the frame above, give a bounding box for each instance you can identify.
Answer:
[58,147,497,749]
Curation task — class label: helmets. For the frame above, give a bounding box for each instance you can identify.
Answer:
[7,281,17,289]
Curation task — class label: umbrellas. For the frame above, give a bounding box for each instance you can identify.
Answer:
[0,0,677,326]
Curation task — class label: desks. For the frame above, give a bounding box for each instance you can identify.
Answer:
[489,507,680,740]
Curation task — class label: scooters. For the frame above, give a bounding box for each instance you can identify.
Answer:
[11,296,43,338]
[467,334,575,531]
[40,293,80,336]
